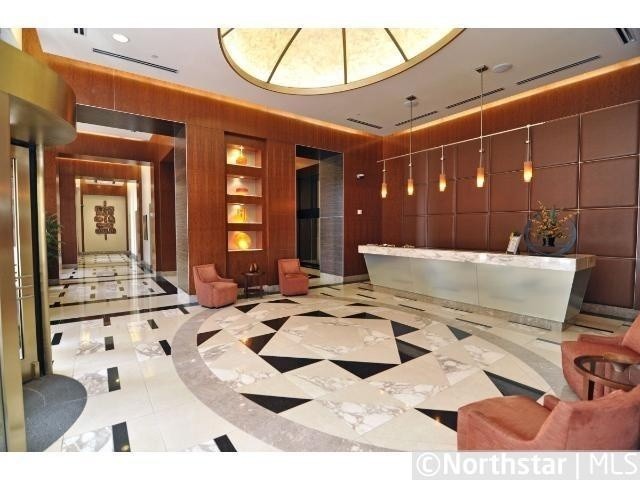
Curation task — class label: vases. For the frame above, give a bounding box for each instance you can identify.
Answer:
[540,241,560,253]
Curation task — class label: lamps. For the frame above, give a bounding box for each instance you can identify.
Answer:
[214,27,467,99]
[377,124,535,199]
[404,63,489,198]
[223,129,268,255]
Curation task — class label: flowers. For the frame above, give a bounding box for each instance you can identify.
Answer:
[527,198,580,242]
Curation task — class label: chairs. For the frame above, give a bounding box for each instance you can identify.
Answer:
[192,261,240,311]
[455,312,640,451]
[275,257,312,297]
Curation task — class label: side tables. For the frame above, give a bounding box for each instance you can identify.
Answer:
[241,270,268,299]
